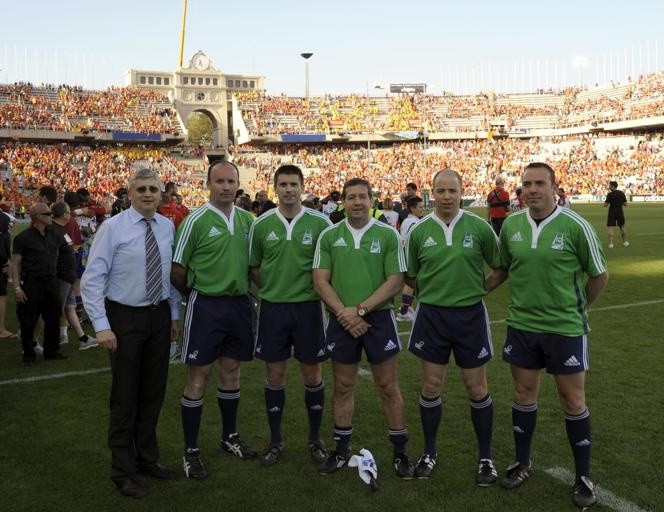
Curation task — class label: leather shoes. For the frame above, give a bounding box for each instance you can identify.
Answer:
[111,476,147,499]
[135,459,179,480]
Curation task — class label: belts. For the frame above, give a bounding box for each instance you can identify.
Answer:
[125,307,162,311]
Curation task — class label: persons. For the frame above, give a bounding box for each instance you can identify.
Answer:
[248,165,334,466]
[499,162,609,507]
[1,69,663,247]
[311,178,417,480]
[1,187,128,360]
[405,169,508,487]
[169,158,257,481]
[80,168,174,498]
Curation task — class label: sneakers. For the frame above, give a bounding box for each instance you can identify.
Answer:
[306,439,329,463]
[318,450,351,475]
[218,432,258,460]
[572,472,596,506]
[500,458,533,490]
[396,313,413,321]
[260,437,285,465]
[184,448,209,482]
[412,452,439,481]
[391,454,412,480]
[475,458,498,486]
[0,318,101,363]
[398,304,414,314]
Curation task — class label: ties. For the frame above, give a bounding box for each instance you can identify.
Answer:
[143,218,163,307]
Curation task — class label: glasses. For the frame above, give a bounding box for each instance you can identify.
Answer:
[34,211,55,216]
